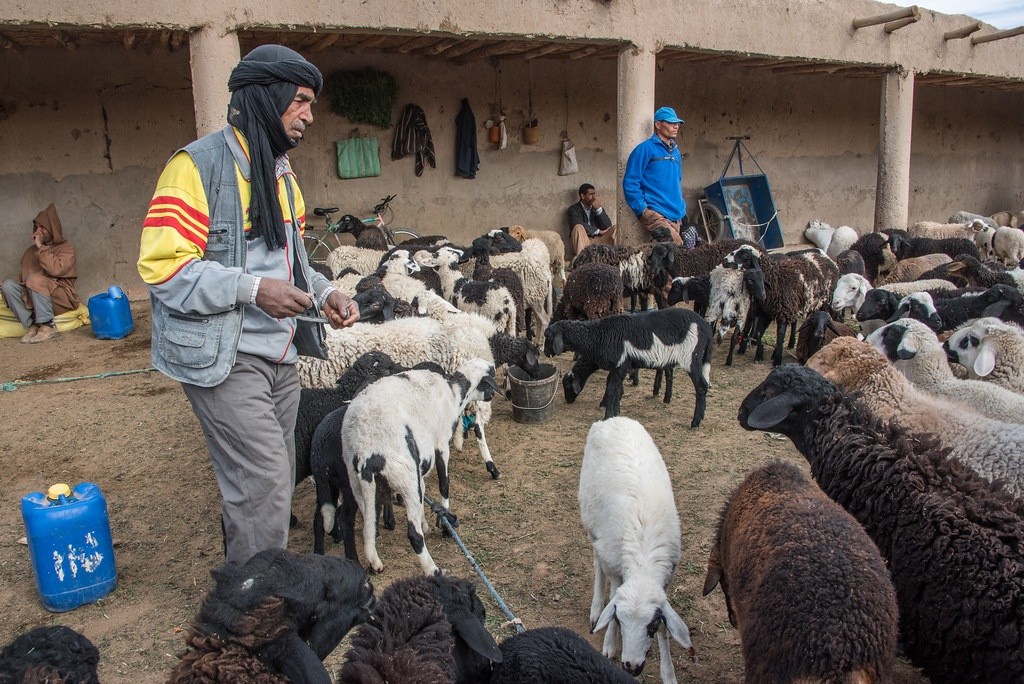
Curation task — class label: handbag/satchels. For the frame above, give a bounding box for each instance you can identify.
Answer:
[336,128,380,179]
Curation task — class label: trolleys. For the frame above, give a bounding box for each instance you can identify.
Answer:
[691,135,785,253]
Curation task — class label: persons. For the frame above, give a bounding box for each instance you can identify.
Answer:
[623,106,689,248]
[566,183,617,259]
[137,43,363,566]
[0,202,81,344]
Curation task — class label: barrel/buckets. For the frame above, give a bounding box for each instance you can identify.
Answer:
[502,362,561,424]
[88,286,135,340]
[21,481,119,613]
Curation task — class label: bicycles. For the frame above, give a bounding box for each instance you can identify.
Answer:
[303,194,423,276]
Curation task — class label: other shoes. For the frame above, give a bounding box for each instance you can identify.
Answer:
[30,323,59,343]
[21,325,39,343]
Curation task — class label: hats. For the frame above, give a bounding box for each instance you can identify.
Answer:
[654,106,685,123]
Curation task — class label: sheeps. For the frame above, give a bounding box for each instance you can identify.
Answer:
[342,356,505,577]
[578,414,695,684]
[831,272,958,335]
[864,318,1023,427]
[493,626,641,684]
[856,284,1024,335]
[736,361,1024,684]
[313,361,446,561]
[292,214,567,480]
[805,336,1023,499]
[827,211,1023,295]
[334,574,504,684]
[0,625,101,684]
[166,547,377,684]
[702,462,899,684]
[549,238,839,365]
[542,306,713,430]
[795,310,857,365]
[289,350,397,529]
